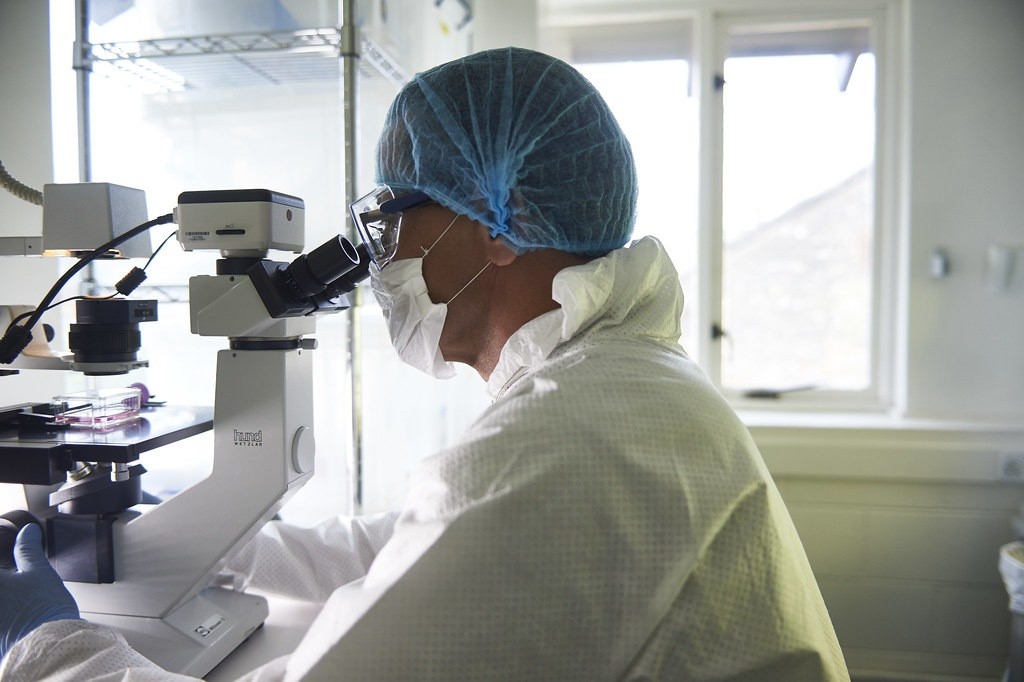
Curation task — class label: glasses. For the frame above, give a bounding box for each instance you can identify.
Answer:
[349,183,436,272]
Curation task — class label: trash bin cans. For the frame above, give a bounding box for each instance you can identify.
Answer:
[994,538,1024,682]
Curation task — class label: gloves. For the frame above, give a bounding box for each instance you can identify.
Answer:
[0,524,80,664]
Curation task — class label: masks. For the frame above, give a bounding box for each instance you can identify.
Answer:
[369,214,492,380]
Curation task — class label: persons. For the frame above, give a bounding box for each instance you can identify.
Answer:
[0,45,851,682]
